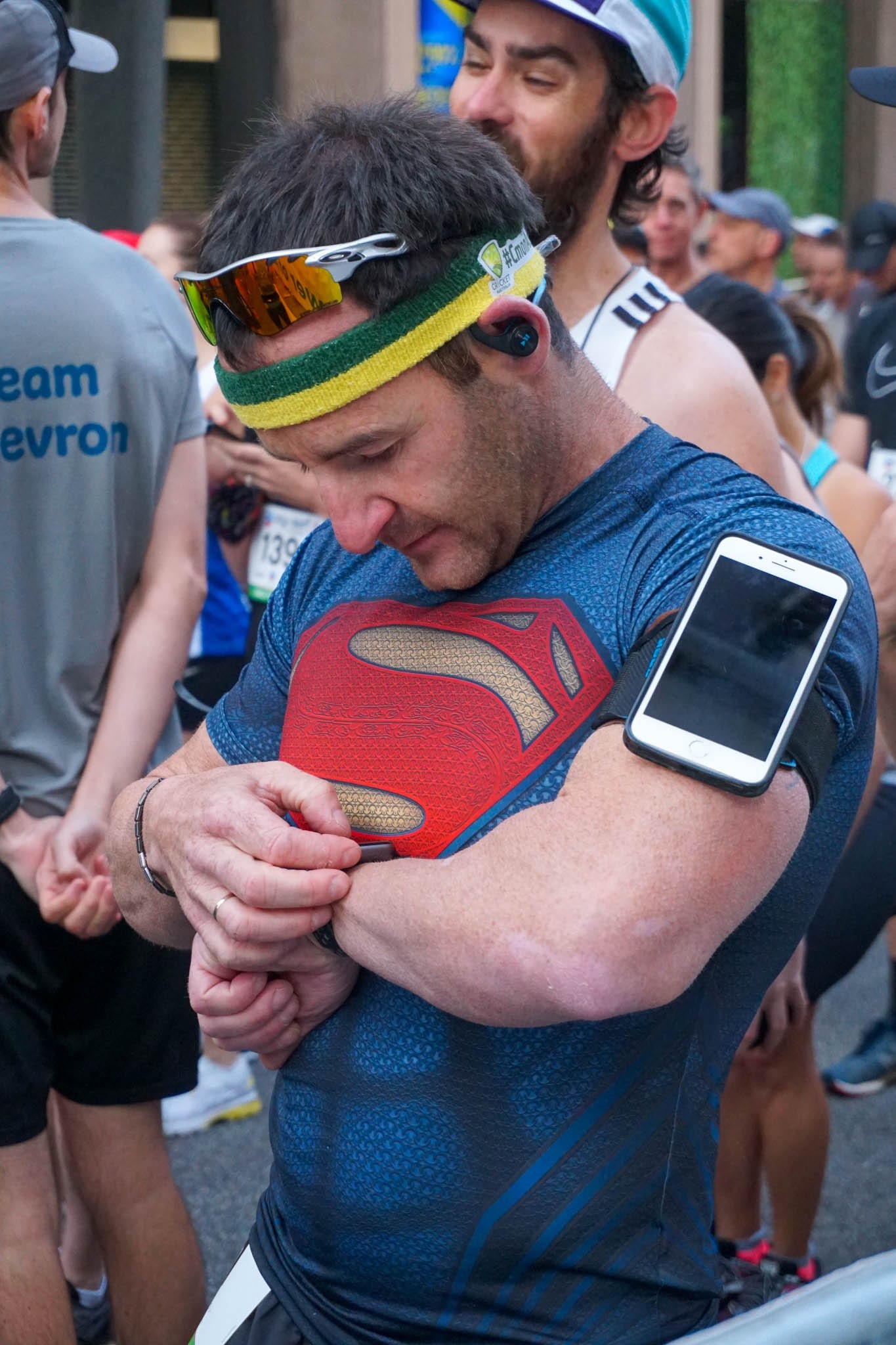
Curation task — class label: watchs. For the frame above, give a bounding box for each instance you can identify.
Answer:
[313,839,397,957]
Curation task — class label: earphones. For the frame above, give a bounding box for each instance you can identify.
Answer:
[469,320,539,357]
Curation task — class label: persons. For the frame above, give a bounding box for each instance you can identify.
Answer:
[676,274,887,1314]
[102,64,894,1138]
[254,271,292,330]
[2,0,210,1345]
[100,94,877,1343]
[438,0,791,507]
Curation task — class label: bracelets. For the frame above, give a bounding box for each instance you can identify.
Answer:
[0,784,23,826]
[134,773,198,899]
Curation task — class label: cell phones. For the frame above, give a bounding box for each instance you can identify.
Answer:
[623,529,855,798]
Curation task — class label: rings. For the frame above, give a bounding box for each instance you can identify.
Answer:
[213,893,234,924]
[244,476,252,487]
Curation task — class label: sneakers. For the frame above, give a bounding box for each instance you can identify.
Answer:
[822,1015,895,1097]
[709,1219,824,1323]
[160,1047,263,1135]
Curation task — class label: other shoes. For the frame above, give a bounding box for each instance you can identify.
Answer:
[63,1266,115,1345]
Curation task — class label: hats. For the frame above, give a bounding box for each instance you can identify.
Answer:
[0,0,121,110]
[437,0,695,96]
[845,203,896,269]
[788,212,841,239]
[702,186,793,243]
[848,64,896,108]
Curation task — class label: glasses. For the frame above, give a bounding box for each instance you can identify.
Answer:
[172,228,437,347]
